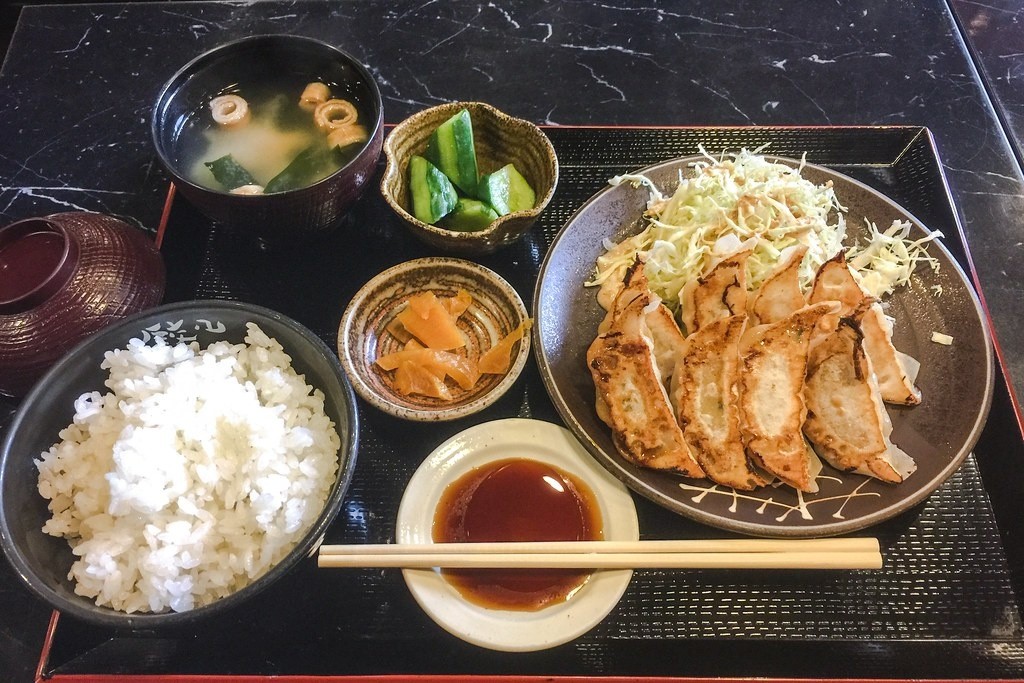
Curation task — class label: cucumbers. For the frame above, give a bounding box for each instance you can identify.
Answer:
[408,108,536,233]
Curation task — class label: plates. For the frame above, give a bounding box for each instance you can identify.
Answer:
[396,418,639,653]
[532,153,996,538]
[338,257,530,421]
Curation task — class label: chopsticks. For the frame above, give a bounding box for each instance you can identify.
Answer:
[318,536,883,570]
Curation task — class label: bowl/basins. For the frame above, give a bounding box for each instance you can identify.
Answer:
[0,299,358,634]
[381,101,559,258]
[149,34,385,246]
[0,210,166,404]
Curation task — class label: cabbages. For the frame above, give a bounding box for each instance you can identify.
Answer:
[582,144,952,345]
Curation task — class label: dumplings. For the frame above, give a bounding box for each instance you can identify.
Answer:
[588,245,922,492]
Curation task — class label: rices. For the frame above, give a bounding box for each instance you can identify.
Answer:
[34,321,343,614]
